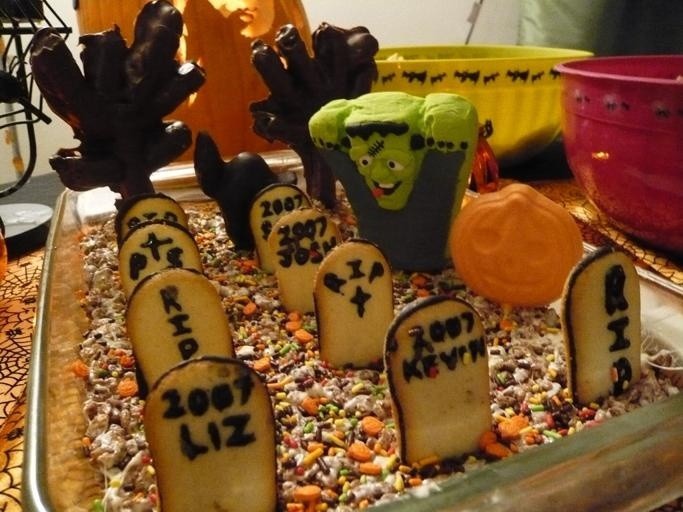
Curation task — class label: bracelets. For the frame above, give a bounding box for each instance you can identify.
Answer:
[370,43,596,185]
[552,55,683,255]
